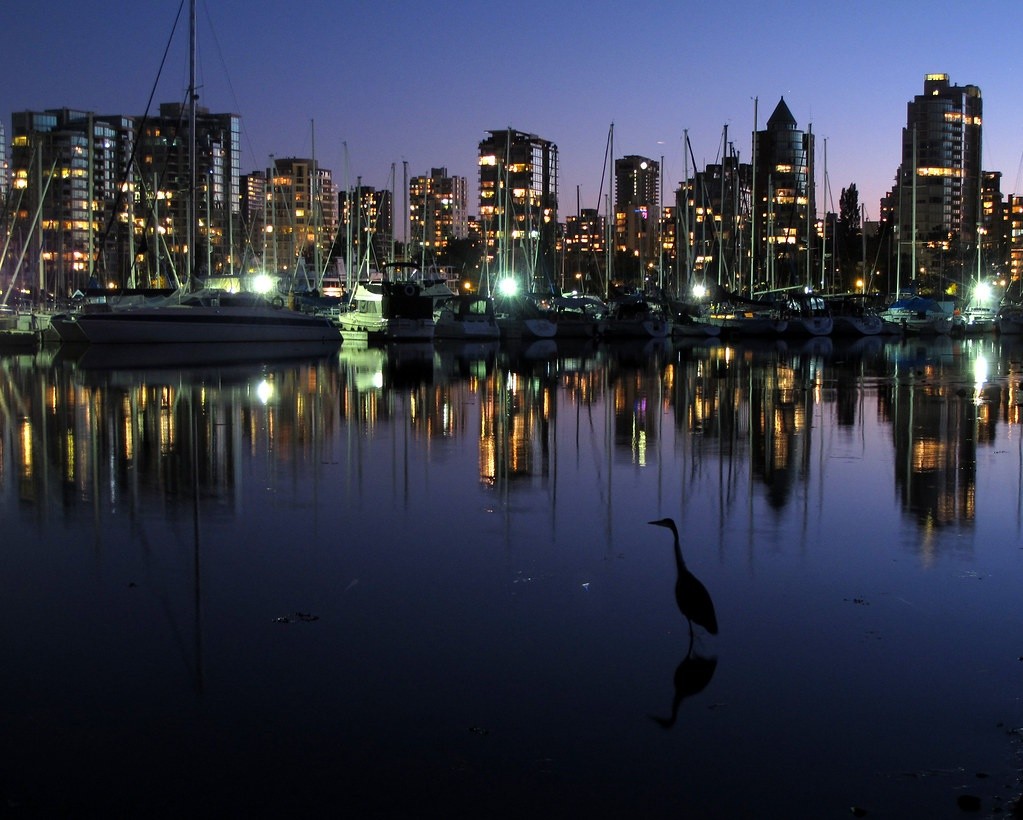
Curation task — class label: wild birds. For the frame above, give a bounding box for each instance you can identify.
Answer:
[647,638,719,729]
[647,517,719,638]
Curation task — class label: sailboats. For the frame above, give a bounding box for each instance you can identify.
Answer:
[0,0,345,365]
[279,95,1023,340]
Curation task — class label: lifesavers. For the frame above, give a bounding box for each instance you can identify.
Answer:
[271,295,284,311]
[405,285,415,296]
